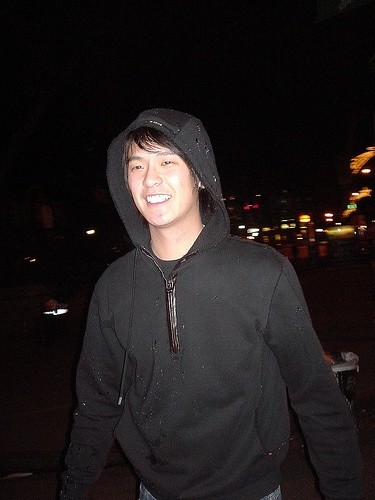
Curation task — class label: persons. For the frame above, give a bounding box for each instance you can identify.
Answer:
[55,103,369,500]
